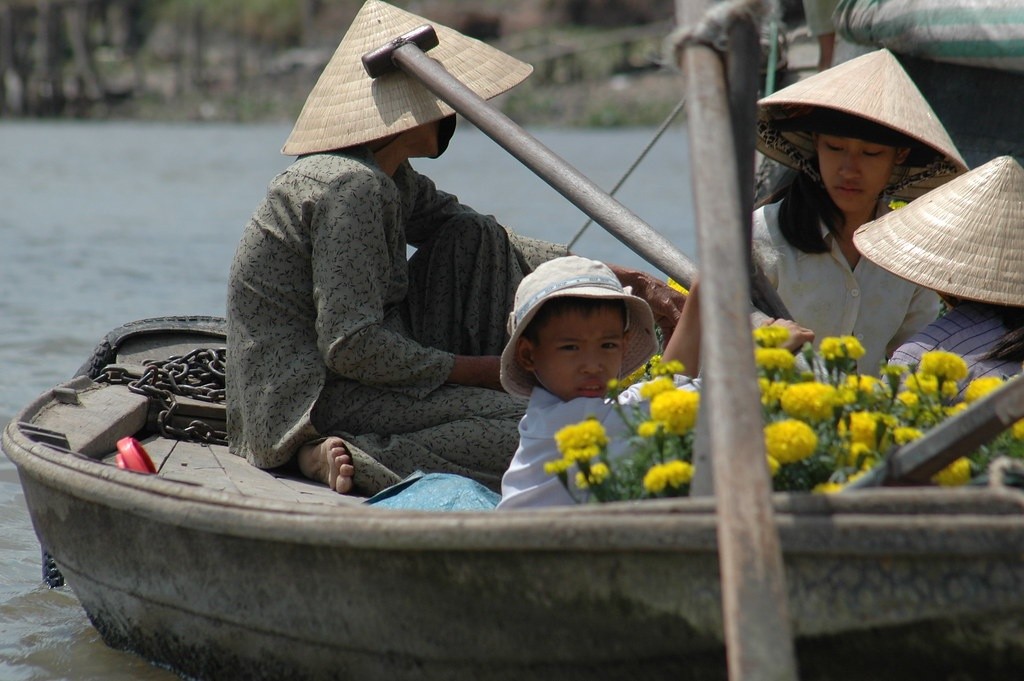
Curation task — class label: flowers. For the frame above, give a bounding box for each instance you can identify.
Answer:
[542,324,1024,494]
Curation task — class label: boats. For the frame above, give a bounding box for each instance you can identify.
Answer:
[0,315,1023,681]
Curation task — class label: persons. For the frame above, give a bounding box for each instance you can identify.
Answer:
[802,0,839,72]
[495,255,814,512]
[851,155,1024,408]
[227,0,686,495]
[749,48,970,381]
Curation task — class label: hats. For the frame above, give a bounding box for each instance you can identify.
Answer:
[281,0,534,156]
[500,255,659,400]
[854,155,1024,307]
[756,46,970,200]
